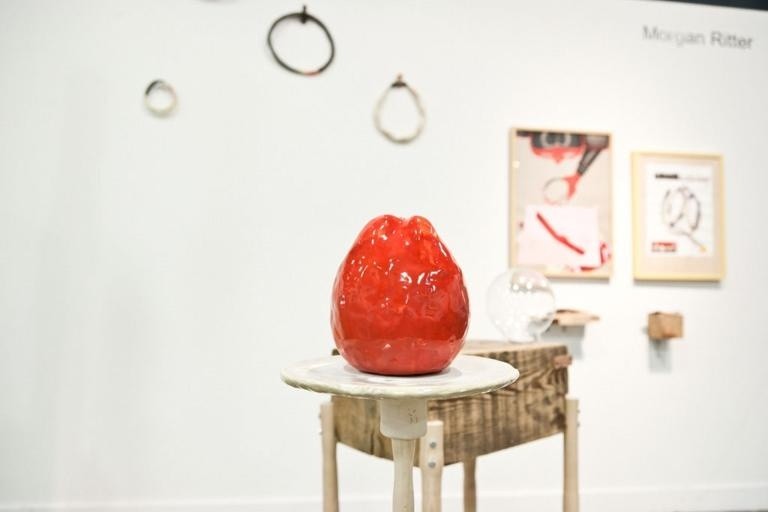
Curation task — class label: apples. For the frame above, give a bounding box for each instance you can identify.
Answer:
[330,214,471,378]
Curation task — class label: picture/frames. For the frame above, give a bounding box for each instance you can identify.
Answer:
[504,125,617,282]
[629,149,725,284]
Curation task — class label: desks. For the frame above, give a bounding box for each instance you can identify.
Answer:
[278,350,520,511]
[319,342,581,511]
